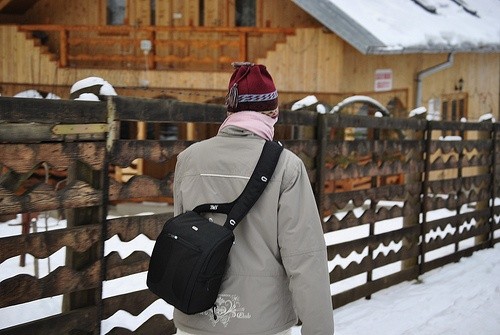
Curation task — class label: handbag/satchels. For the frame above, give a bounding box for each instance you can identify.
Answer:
[147,208,234,315]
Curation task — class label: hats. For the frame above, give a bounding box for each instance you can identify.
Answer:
[224,61,280,120]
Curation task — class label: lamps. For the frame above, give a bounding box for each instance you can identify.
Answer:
[140,39,152,86]
[454,78,464,91]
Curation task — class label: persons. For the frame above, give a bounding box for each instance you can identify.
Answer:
[172,60,336,335]
